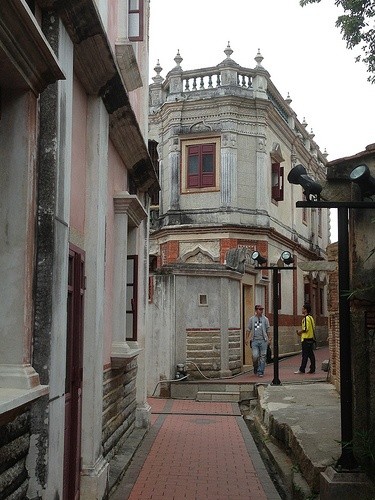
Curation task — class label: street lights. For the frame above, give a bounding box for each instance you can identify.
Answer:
[250,250,297,391]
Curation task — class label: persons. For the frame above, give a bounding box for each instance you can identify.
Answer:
[245,304,272,377]
[294,304,317,375]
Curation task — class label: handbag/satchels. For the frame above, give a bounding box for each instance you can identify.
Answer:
[313,339,317,350]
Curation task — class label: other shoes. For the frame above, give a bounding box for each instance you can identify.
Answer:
[254,368,257,374]
[259,372,263,377]
[307,371,311,373]
[293,370,305,374]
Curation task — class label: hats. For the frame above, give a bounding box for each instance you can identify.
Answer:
[255,304,264,309]
